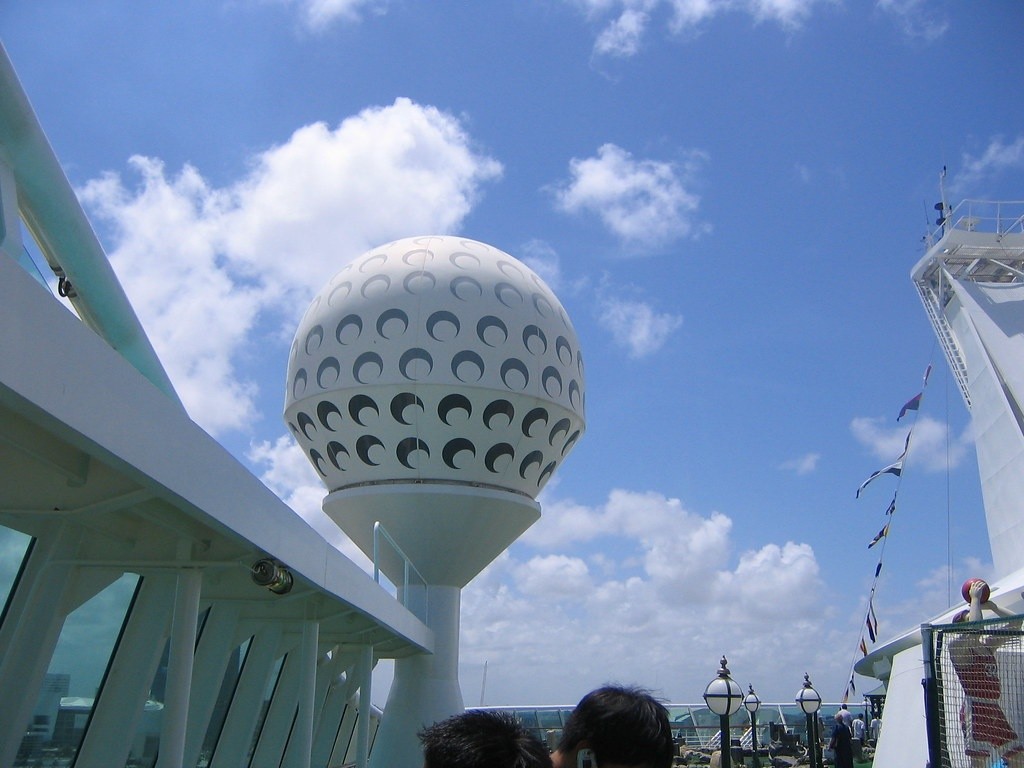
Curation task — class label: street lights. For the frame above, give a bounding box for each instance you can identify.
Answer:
[701,654,745,768]
[743,682,762,768]
[795,672,823,768]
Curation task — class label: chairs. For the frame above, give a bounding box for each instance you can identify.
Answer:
[674,751,692,766]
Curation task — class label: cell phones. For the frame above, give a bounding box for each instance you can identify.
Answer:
[576,749,598,768]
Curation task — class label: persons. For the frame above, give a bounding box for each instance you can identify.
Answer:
[413,710,556,768]
[947,581,1024,768]
[541,685,675,768]
[816,704,882,768]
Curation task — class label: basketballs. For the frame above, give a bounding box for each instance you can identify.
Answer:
[961,577,990,604]
